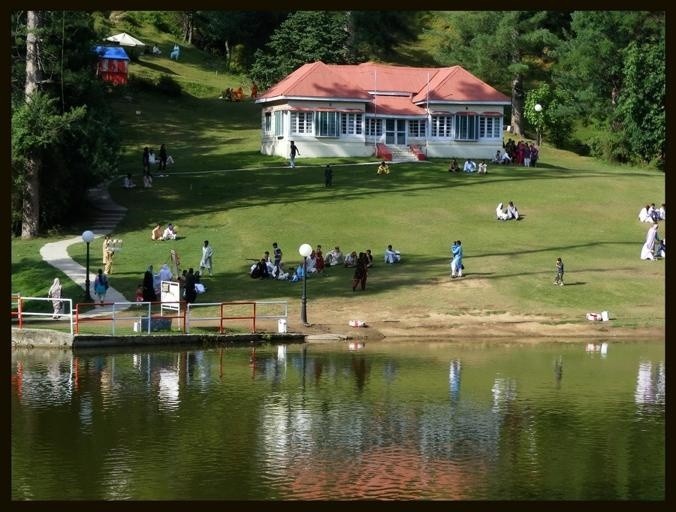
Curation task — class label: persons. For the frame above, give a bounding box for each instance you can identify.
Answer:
[218,80,259,103]
[448,159,461,172]
[48,278,62,319]
[505,201,519,221]
[169,43,180,61]
[638,202,666,262]
[450,239,464,278]
[152,44,161,55]
[477,159,488,175]
[384,245,400,265]
[102,234,114,274]
[463,158,476,172]
[451,242,457,257]
[257,242,373,293]
[121,142,175,190]
[553,257,565,287]
[496,201,510,221]
[135,263,206,305]
[289,141,301,169]
[94,269,109,308]
[325,164,334,188]
[198,239,215,277]
[376,161,391,175]
[162,223,178,241]
[491,136,540,167]
[151,223,164,240]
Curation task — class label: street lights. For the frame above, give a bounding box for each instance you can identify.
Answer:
[296,240,316,333]
[78,228,94,308]
[295,345,319,429]
[532,100,544,151]
